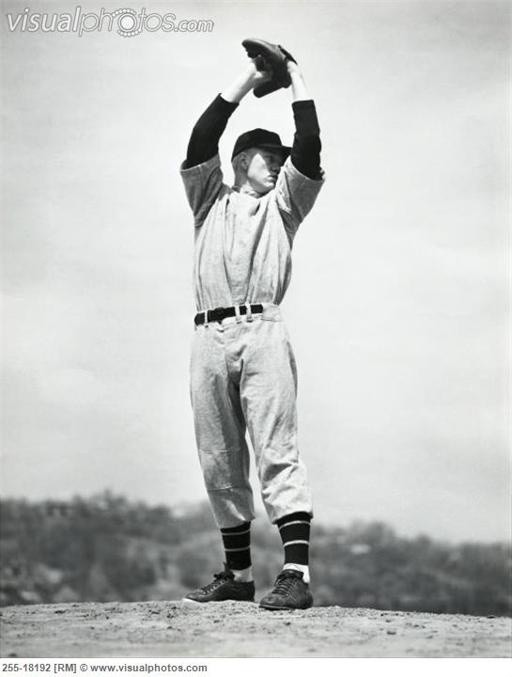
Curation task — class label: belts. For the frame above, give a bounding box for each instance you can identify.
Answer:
[195,304,263,325]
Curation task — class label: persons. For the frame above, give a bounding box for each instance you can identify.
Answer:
[180,38,325,608]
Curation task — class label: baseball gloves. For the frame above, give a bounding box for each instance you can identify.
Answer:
[243,38,297,97]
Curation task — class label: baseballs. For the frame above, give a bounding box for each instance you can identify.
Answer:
[256,52,275,71]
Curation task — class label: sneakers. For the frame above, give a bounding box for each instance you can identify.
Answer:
[185,571,255,602]
[258,569,313,610]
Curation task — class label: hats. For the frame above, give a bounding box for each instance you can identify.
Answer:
[231,128,292,161]
